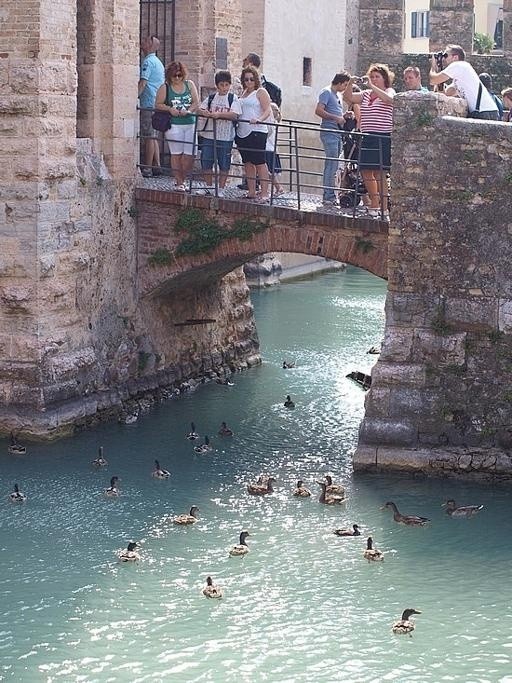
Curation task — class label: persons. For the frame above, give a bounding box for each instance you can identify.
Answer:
[235,66,272,199]
[137,36,165,177]
[315,42,512,217]
[237,54,260,191]
[156,61,200,192]
[195,71,243,198]
[261,83,285,199]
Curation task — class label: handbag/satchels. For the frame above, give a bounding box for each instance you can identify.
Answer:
[150,111,174,133]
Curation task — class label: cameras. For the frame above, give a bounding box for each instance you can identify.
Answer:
[356,77,364,83]
[427,51,443,66]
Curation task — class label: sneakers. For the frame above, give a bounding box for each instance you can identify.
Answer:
[140,161,164,178]
[322,193,392,224]
[237,180,286,203]
[173,183,192,192]
[214,187,225,198]
[197,185,214,195]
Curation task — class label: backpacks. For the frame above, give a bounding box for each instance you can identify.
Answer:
[261,75,283,110]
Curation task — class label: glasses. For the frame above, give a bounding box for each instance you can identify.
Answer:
[171,73,184,78]
[243,76,255,82]
[442,52,460,58]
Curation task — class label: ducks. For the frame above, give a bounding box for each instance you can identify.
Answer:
[216,372,231,385]
[393,608,422,639]
[91,408,277,499]
[7,439,26,455]
[118,542,141,566]
[284,395,295,408]
[283,360,297,368]
[8,482,27,506]
[292,477,484,564]
[202,576,223,601]
[172,504,201,525]
[228,531,252,560]
